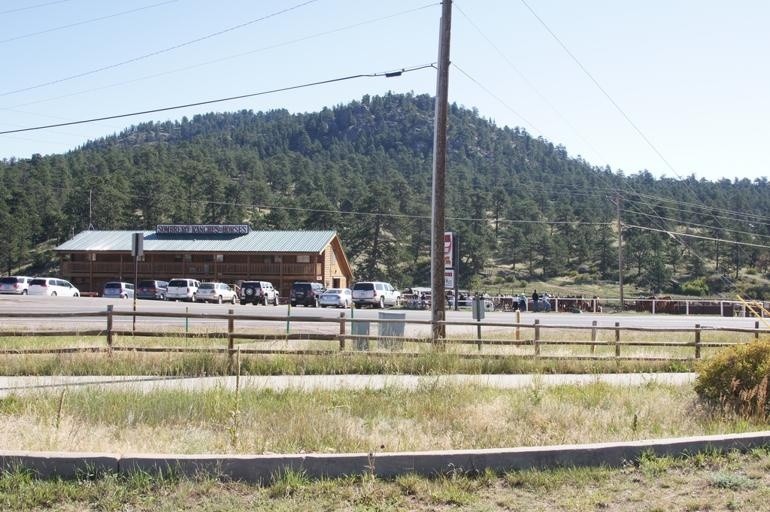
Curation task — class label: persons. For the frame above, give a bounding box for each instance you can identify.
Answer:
[401,287,551,313]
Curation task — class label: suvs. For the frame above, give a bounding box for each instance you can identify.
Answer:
[239,281,280,306]
[290,281,327,307]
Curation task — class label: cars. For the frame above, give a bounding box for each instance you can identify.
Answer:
[0,275,80,298]
[104,277,238,305]
[319,282,402,308]
